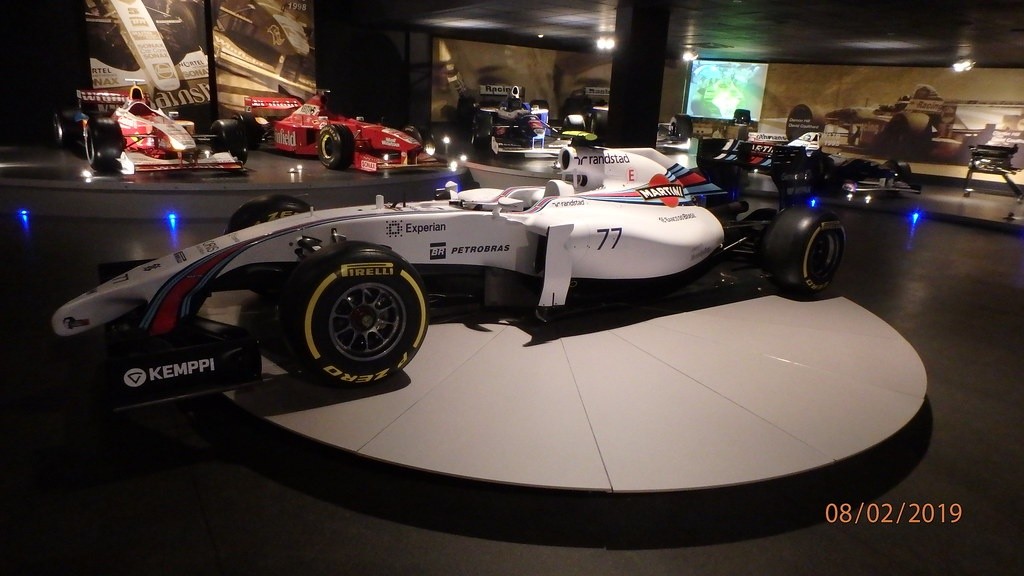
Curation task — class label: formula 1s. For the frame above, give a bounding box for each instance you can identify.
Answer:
[782,82,1024,170]
[44,129,847,395]
[231,86,451,176]
[451,75,580,160]
[560,83,696,151]
[52,80,251,176]
[83,1,208,100]
[212,0,321,98]
[699,128,923,209]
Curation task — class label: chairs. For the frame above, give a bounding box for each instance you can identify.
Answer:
[509,178,576,218]
[316,110,343,122]
[499,84,532,119]
[136,112,164,125]
[813,148,849,171]
